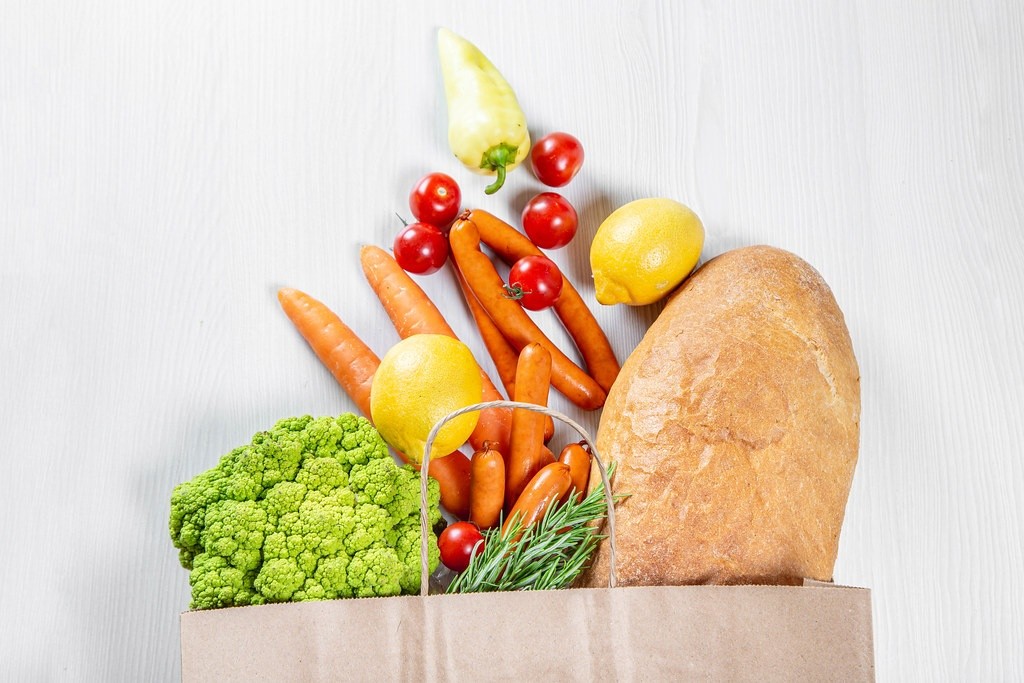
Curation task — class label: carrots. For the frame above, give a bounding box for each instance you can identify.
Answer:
[361,245,556,475]
[277,287,475,520]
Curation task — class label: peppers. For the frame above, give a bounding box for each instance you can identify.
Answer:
[438,27,529,195]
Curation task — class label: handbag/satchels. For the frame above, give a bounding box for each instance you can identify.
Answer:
[180,402,875,683]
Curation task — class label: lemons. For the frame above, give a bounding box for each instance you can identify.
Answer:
[370,333,480,467]
[588,198,705,306]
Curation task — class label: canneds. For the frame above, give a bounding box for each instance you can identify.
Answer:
[575,244,863,586]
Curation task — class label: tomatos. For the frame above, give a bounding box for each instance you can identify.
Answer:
[507,254,563,310]
[521,192,578,251]
[393,223,449,275]
[408,172,461,227]
[436,521,485,571]
[532,131,585,188]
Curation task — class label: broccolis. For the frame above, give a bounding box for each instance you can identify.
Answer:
[167,413,444,610]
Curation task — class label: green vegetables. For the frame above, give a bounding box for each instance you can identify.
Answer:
[441,462,632,594]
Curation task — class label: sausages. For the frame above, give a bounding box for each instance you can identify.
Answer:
[444,210,622,544]
